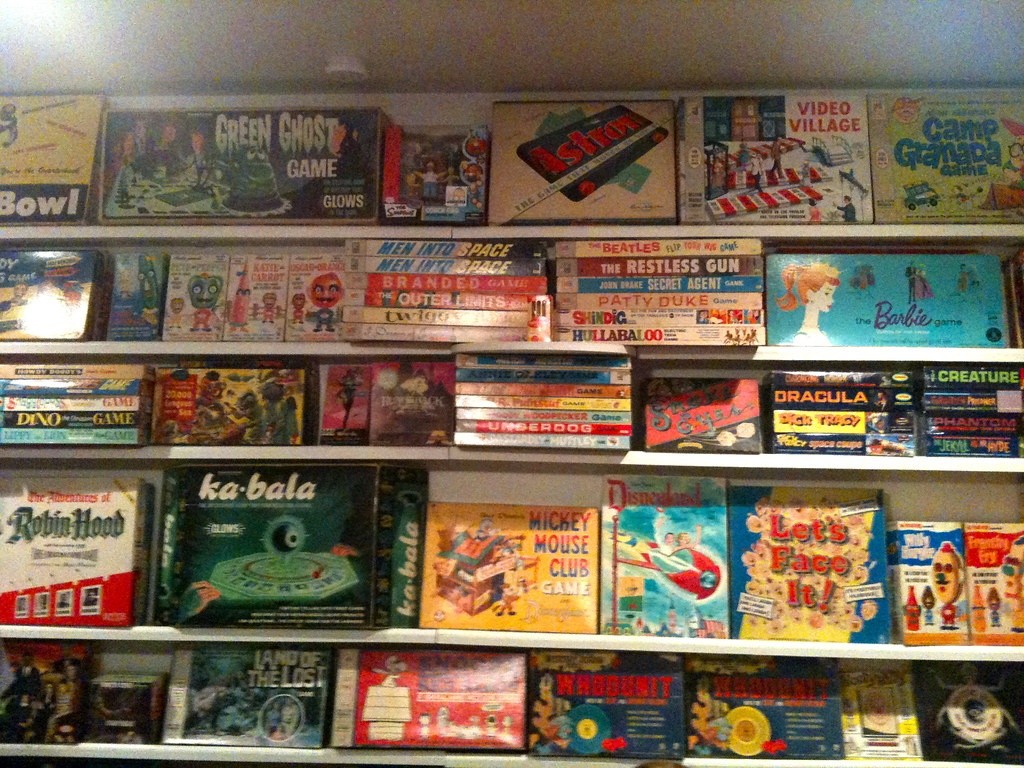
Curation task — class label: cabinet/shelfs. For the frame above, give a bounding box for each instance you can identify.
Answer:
[0,219,1024,768]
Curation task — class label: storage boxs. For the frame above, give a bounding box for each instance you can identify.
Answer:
[380,123,490,225]
[154,465,424,627]
[99,106,390,224]
[675,98,875,223]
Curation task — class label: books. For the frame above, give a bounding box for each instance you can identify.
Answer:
[0,478,155,627]
[160,642,924,763]
[418,474,1024,648]
[163,238,768,347]
[1,354,1024,459]
